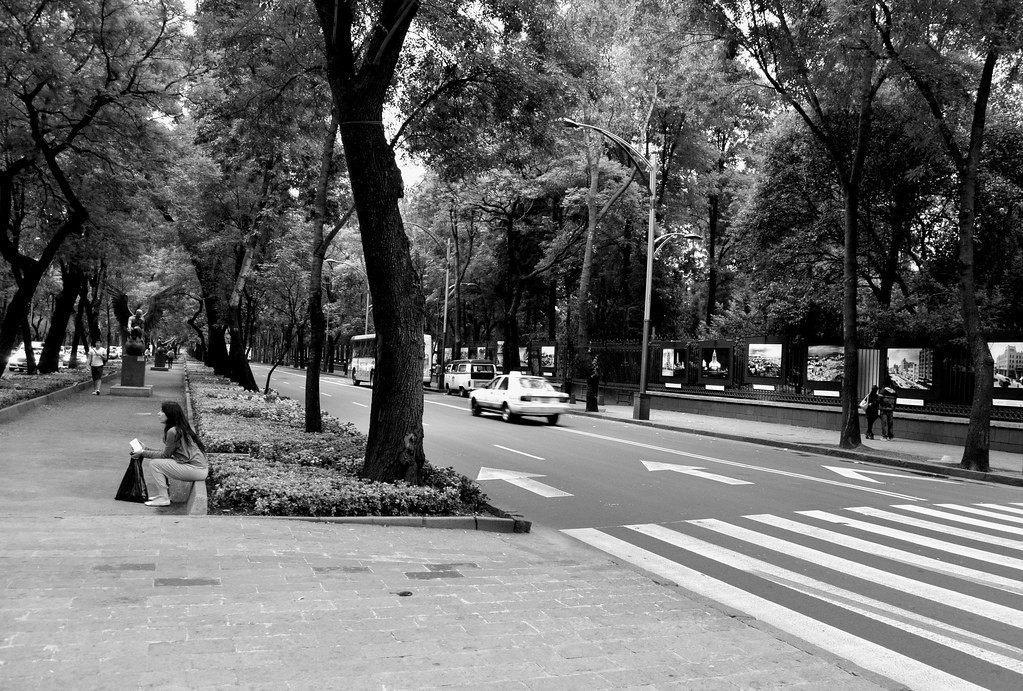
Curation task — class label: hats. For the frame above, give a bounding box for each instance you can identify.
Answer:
[872,385,878,389]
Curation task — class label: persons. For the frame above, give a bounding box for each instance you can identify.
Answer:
[126,309,145,344]
[865,385,880,439]
[144,348,151,362]
[166,342,175,369]
[130,401,209,506]
[869,381,897,441]
[86,339,107,395]
[432,361,442,383]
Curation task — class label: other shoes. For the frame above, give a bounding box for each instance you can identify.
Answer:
[92,392,96,395]
[144,498,171,506]
[867,433,873,439]
[149,495,158,500]
[866,432,869,439]
[96,390,100,395]
[889,433,896,440]
[880,437,887,442]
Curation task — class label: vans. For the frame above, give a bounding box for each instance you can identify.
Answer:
[442,360,499,398]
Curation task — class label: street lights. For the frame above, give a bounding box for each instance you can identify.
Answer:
[404,219,479,389]
[325,258,373,335]
[554,113,703,422]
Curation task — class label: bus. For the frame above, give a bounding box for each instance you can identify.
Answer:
[346,332,433,388]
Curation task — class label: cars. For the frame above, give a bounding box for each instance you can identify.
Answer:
[469,371,571,426]
[6,340,121,372]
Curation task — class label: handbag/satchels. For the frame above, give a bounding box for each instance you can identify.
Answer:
[114,452,149,504]
[860,394,869,411]
